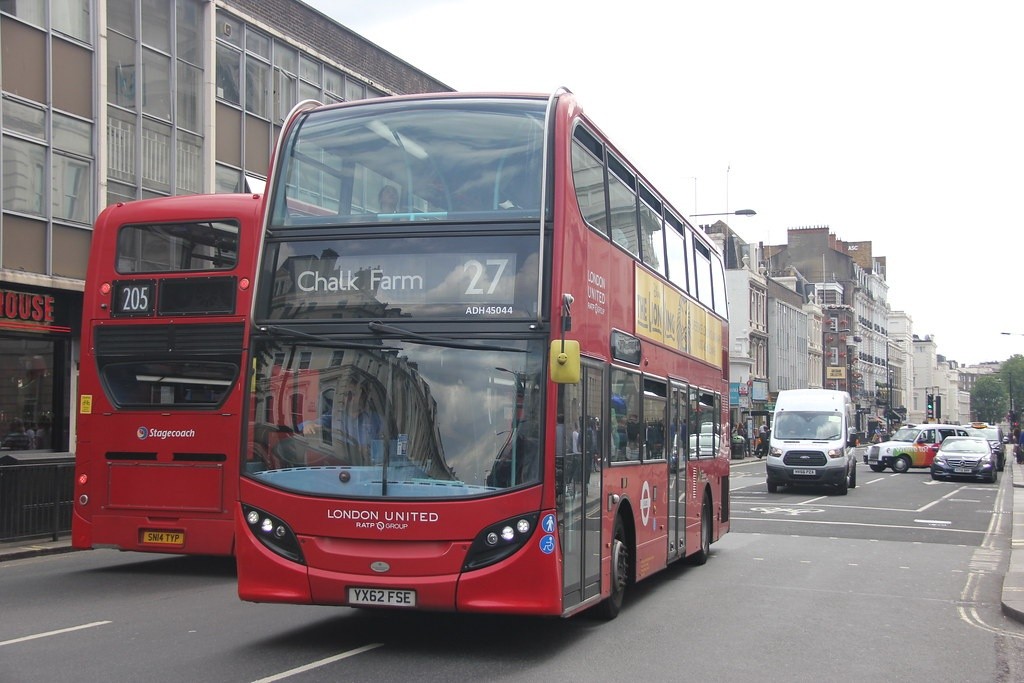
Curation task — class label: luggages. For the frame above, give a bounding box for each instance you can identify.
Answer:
[731,444,744,459]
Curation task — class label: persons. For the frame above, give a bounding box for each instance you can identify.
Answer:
[759,421,769,442]
[4,415,51,450]
[738,422,746,437]
[297,383,390,446]
[374,186,408,222]
[556,415,690,511]
[731,425,737,437]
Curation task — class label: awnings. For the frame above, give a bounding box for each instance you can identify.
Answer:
[873,417,887,424]
[893,408,907,413]
[889,410,901,419]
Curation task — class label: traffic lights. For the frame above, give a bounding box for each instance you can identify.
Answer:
[927,394,933,418]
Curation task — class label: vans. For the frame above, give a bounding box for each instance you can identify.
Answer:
[766,389,859,495]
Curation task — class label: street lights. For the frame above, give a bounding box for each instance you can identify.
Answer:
[494,367,519,487]
[823,329,850,389]
[889,370,893,425]
[1001,332,1024,336]
[689,209,756,217]
[993,371,1012,432]
[886,330,904,429]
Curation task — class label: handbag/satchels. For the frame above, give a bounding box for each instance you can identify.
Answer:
[733,435,745,444]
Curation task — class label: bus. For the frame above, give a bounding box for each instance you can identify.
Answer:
[72,194,338,556]
[236,86,730,617]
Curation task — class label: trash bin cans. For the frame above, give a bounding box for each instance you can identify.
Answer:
[0,453,76,543]
[731,435,744,459]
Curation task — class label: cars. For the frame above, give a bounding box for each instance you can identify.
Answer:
[690,433,720,460]
[931,436,998,483]
[968,429,1006,472]
[863,424,972,472]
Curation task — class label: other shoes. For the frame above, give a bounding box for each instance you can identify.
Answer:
[757,456,762,458]
